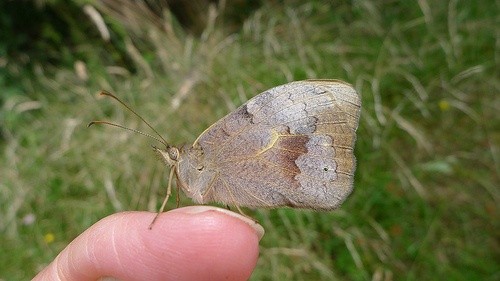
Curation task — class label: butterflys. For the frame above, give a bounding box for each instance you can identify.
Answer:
[88,77,361,231]
